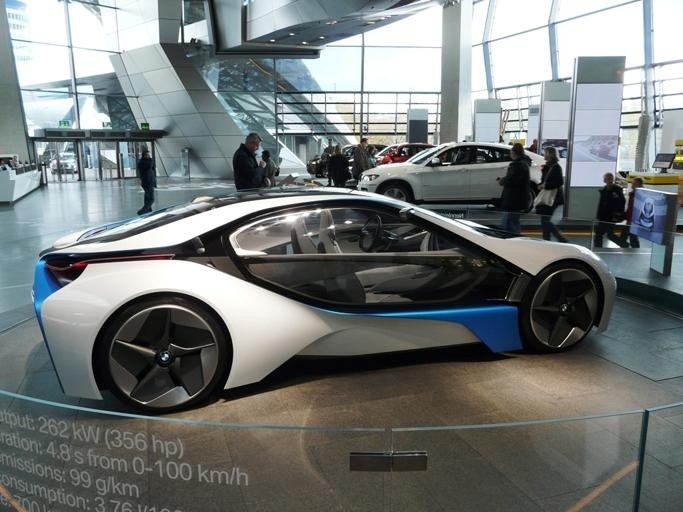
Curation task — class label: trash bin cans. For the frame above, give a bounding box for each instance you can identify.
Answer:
[38,161,47,185]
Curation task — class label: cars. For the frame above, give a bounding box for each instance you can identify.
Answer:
[305,137,547,216]
[588,144,610,159]
[540,139,566,158]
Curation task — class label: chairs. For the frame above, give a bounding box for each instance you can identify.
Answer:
[289,210,365,307]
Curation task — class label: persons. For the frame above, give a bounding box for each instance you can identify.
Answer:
[535,145,569,242]
[323,135,377,188]
[621,178,645,248]
[231,131,273,189]
[136,149,158,216]
[260,147,280,187]
[530,138,538,153]
[498,142,533,239]
[594,172,630,248]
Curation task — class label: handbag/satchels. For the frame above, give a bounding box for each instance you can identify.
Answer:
[533,188,558,210]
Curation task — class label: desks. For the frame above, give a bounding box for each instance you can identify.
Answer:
[626,173,682,229]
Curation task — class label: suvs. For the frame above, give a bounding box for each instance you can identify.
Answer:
[49,152,77,175]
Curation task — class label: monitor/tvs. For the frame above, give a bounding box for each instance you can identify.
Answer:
[652,154,677,172]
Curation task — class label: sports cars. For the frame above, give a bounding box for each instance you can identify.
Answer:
[24,184,620,417]
